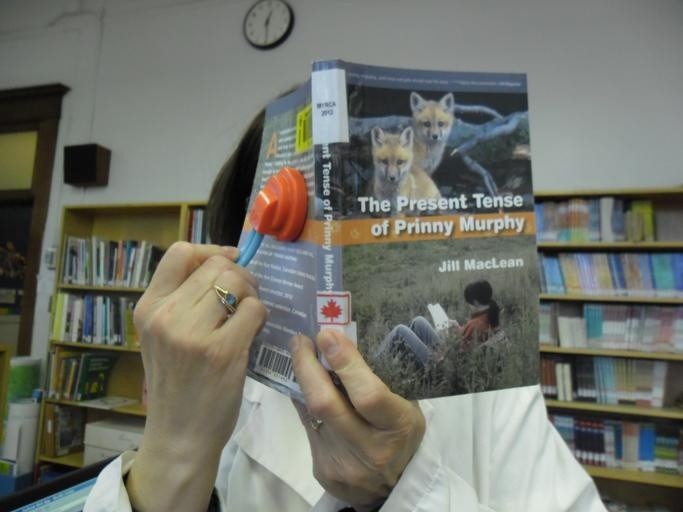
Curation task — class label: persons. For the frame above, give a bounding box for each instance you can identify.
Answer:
[373,278,500,373]
[80,80,609,511]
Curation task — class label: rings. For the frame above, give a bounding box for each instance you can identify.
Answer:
[308,417,322,430]
[213,284,237,314]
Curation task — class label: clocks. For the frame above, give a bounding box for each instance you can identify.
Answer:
[241,0,294,50]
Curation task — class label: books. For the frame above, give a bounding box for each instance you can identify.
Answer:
[230,58,543,407]
[538,302,682,355]
[541,357,682,407]
[548,412,682,477]
[39,234,171,459]
[537,251,682,298]
[426,303,451,332]
[535,199,655,242]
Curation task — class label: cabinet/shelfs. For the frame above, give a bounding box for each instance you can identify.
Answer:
[30,204,208,482]
[531,186,681,511]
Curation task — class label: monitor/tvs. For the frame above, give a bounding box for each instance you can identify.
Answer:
[0,454,127,512]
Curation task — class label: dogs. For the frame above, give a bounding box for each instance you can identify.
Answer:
[409,91,454,176]
[370,124,444,216]
